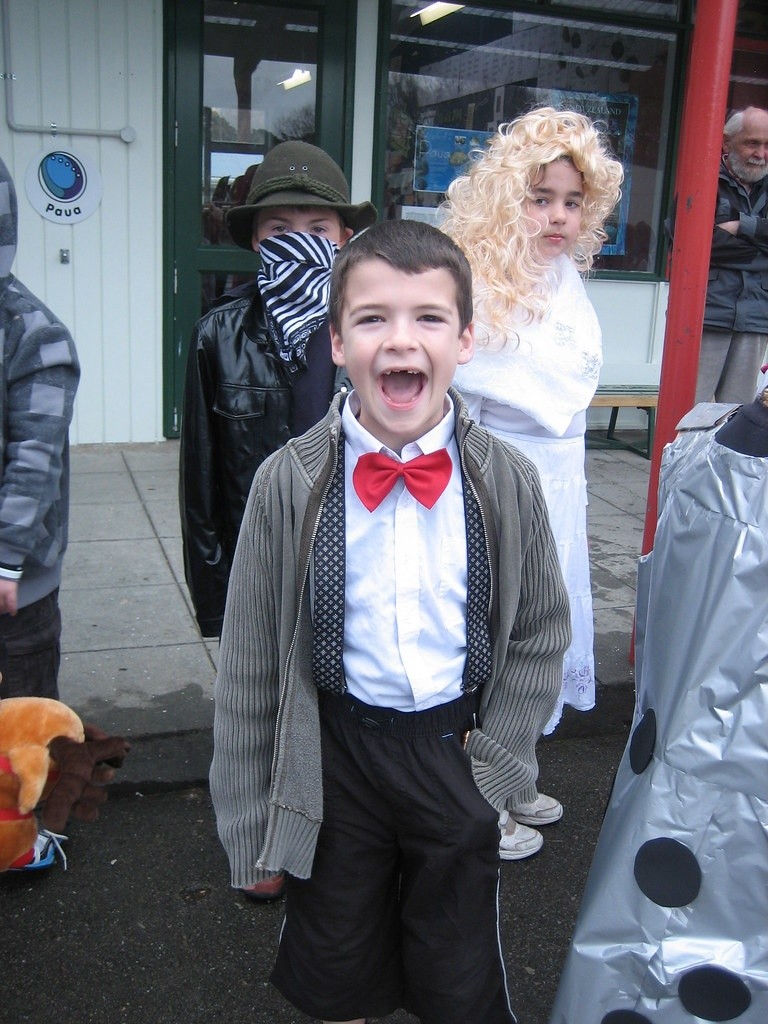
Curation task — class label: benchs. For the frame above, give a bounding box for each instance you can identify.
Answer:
[585,385,660,460]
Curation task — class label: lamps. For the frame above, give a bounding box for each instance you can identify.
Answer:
[275,64,312,91]
[409,0,465,26]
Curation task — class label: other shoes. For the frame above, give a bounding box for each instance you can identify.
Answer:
[240,867,286,898]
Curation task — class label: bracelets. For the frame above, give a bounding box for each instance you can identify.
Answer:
[0,567,23,579]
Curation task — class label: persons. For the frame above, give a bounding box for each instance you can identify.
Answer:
[178,140,379,901]
[439,106,625,860]
[0,157,80,700]
[697,106,768,404]
[562,388,768,1024]
[209,220,571,1023]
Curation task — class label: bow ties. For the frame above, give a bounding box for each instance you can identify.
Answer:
[352,448,453,514]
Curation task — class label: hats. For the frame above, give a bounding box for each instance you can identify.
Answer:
[224,140,377,251]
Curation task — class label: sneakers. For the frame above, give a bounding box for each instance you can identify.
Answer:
[510,792,563,826]
[498,810,544,860]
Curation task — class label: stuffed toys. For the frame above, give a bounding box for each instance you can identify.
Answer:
[0,697,129,871]
